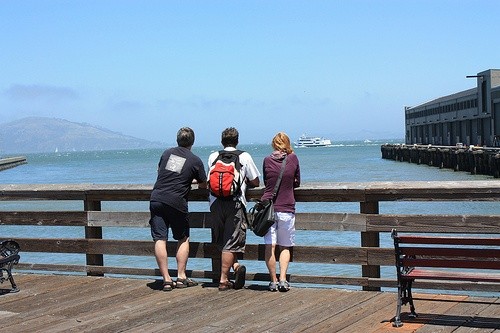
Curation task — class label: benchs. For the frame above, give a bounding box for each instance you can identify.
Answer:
[390,228,500,328]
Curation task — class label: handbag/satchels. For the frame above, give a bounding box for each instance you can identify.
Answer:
[251,199,274,236]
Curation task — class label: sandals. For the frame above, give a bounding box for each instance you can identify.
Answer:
[234,265,247,289]
[162,282,176,291]
[219,282,231,290]
[177,278,198,288]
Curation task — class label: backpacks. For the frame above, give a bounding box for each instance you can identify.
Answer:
[209,150,241,197]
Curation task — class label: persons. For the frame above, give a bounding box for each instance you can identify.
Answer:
[206,126,262,290]
[258,132,301,293]
[149,126,207,291]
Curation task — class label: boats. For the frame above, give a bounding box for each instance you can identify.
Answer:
[293,132,332,148]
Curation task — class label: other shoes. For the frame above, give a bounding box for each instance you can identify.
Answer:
[269,282,278,291]
[279,282,288,291]
